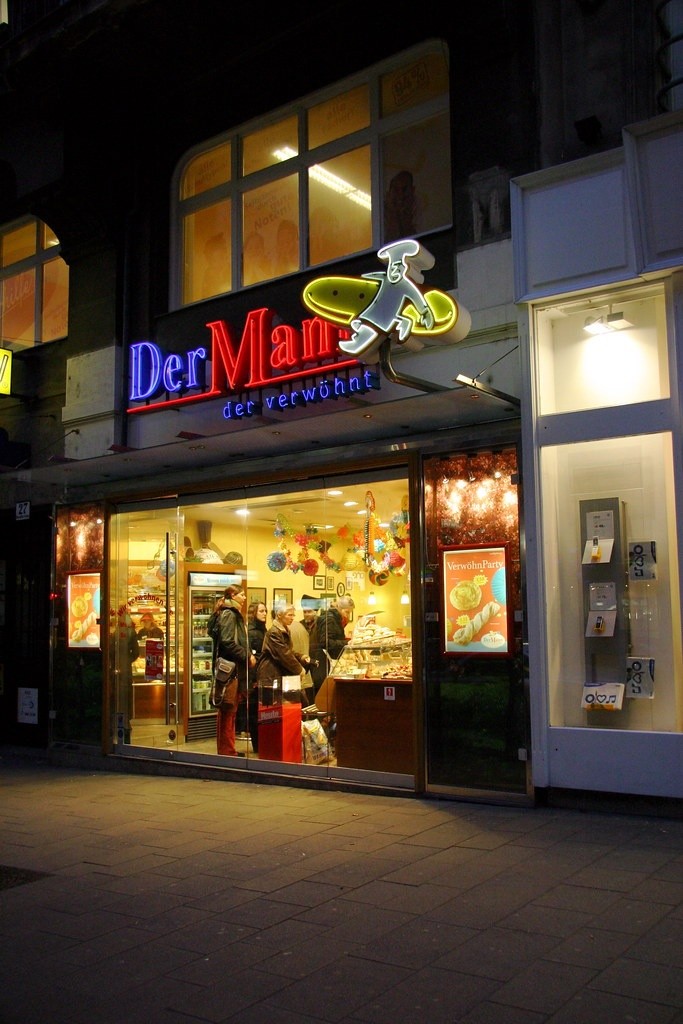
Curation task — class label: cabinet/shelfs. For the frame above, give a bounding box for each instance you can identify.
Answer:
[110,560,184,719]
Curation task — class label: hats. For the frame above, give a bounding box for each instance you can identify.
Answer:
[140,614,153,622]
[301,595,322,611]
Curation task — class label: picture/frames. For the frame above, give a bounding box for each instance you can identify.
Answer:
[246,574,353,621]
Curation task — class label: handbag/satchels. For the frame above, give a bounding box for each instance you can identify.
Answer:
[208,674,237,707]
[302,718,335,764]
[214,657,236,682]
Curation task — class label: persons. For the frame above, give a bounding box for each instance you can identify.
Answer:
[248,601,267,753]
[272,607,316,702]
[111,607,139,745]
[309,597,355,691]
[207,584,257,757]
[257,604,312,679]
[299,594,326,631]
[137,612,164,640]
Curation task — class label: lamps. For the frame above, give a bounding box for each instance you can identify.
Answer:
[581,305,634,334]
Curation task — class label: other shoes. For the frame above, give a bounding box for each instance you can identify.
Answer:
[237,752,246,757]
